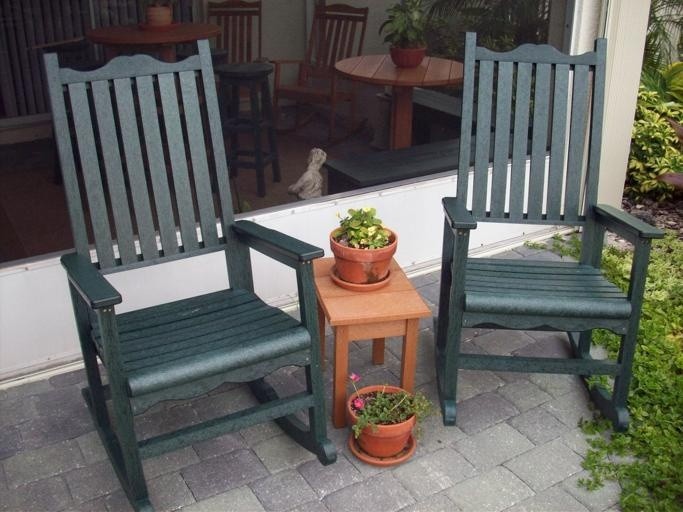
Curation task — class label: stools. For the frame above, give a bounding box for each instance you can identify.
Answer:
[176,49,227,66]
[214,61,282,197]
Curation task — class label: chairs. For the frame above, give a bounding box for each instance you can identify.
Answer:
[269,4,369,143]
[433,30,666,434]
[43,39,338,512]
[206,0,261,103]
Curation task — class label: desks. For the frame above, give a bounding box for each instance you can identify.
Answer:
[312,256,431,429]
[333,55,464,150]
[85,25,221,62]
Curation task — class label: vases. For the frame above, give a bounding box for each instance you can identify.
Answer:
[346,385,418,467]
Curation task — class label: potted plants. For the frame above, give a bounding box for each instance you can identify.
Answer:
[346,373,433,439]
[329,207,398,291]
[147,0,177,28]
[378,0,441,67]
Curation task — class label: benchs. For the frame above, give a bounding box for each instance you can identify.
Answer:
[323,132,532,194]
[372,88,533,150]
